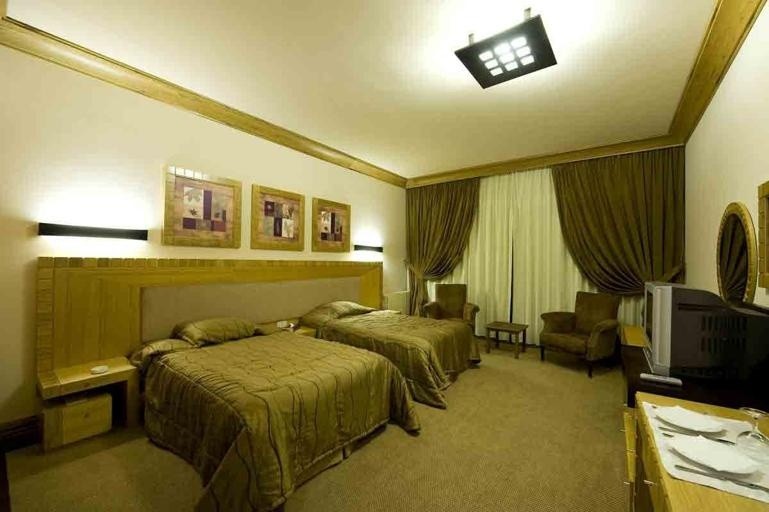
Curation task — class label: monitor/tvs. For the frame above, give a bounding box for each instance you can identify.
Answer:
[643,281,769,379]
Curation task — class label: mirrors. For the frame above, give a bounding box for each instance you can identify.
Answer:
[716,202,758,305]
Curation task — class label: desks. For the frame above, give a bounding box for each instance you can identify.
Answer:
[622,343,768,407]
[484,320,529,360]
[622,324,642,347]
[623,391,769,510]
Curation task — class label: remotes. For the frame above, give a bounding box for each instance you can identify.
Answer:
[640,373,685,386]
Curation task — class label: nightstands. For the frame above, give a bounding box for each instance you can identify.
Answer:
[253,312,316,339]
[34,355,140,429]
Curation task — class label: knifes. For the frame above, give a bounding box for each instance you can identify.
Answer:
[658,426,734,444]
[674,463,769,493]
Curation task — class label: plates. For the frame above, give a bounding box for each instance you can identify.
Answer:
[670,436,758,474]
[655,407,723,434]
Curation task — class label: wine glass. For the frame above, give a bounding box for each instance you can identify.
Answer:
[736,407,769,463]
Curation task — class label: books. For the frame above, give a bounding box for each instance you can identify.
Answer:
[656,404,769,492]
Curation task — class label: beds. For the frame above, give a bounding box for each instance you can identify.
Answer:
[298,274,482,408]
[139,282,419,511]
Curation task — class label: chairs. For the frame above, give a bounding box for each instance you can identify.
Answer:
[424,281,479,334]
[539,289,621,379]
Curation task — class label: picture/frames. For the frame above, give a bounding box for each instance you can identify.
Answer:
[756,181,769,288]
[312,196,351,253]
[162,165,242,251]
[250,185,305,254]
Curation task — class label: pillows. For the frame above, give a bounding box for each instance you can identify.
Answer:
[300,298,376,330]
[170,318,267,349]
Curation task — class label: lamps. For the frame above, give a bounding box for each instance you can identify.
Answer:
[455,6,558,89]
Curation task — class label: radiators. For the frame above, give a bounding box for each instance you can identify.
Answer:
[382,291,410,314]
